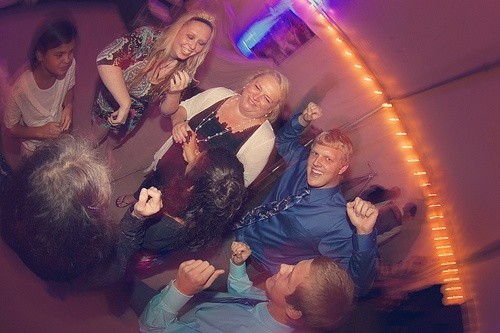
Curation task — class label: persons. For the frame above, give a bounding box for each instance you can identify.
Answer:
[0,135,139,333]
[128,241,356,333]
[3,18,78,160]
[116,69,289,209]
[86,10,216,159]
[116,101,417,296]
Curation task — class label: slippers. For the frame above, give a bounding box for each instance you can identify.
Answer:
[115,195,137,209]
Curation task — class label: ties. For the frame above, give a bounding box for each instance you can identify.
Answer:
[229,189,311,231]
[188,297,269,306]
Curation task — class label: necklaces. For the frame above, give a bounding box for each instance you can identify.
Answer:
[194,96,268,143]
